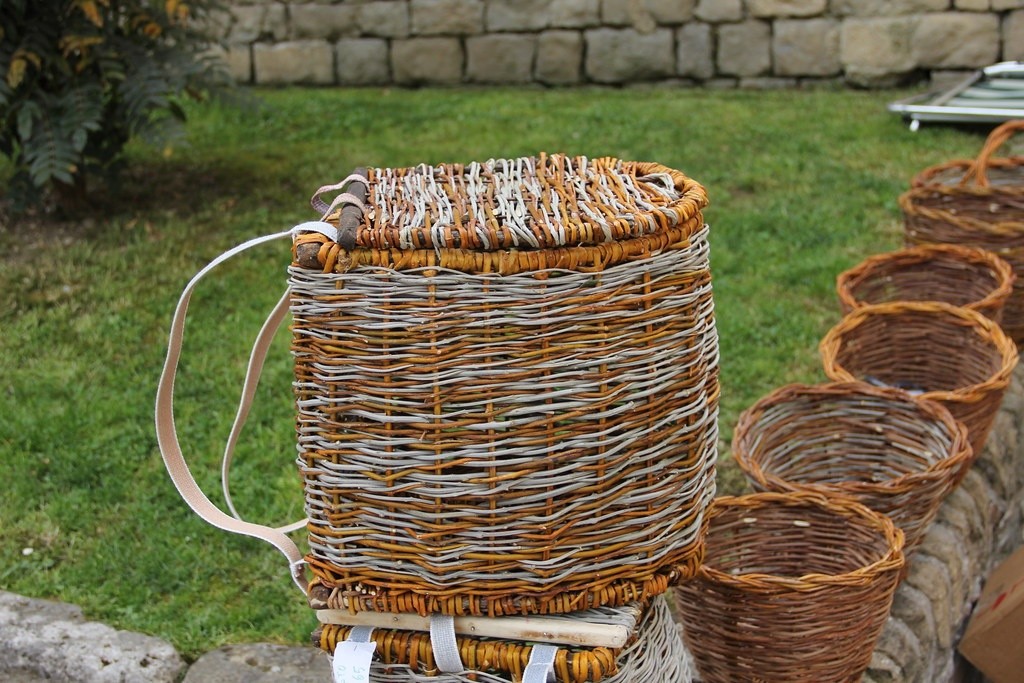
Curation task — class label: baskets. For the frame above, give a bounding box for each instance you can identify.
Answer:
[821,303,1019,494]
[899,186,1024,350]
[156,154,720,610]
[832,244,1013,327]
[730,382,972,592]
[912,120,1024,189]
[668,492,905,683]
[314,595,693,683]
